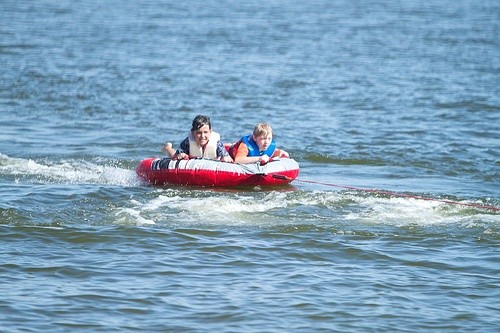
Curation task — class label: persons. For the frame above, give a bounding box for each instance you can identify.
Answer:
[225,123,290,166]
[160,115,235,163]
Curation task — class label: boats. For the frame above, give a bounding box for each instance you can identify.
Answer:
[136,142,300,188]
